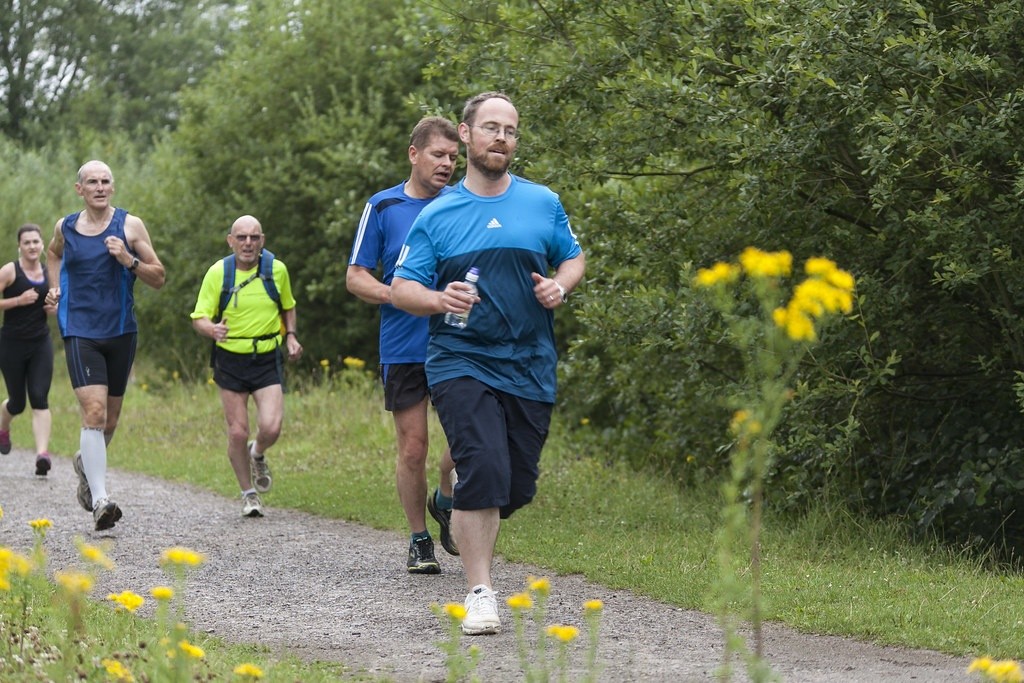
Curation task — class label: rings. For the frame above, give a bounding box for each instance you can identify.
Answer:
[550,294,554,301]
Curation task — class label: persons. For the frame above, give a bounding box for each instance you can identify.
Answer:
[44,160,166,531]
[191,215,304,517]
[0,223,55,476]
[346,116,460,575]
[391,93,586,635]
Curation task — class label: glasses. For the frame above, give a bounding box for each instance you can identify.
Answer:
[231,235,260,241]
[466,124,520,141]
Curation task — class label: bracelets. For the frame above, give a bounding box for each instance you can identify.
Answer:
[16,297,19,305]
[286,331,297,339]
[126,256,139,272]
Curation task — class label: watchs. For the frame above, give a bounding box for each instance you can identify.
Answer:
[555,280,568,304]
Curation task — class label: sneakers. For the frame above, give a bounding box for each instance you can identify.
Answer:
[241,492,264,516]
[428,487,460,556]
[407,536,441,573]
[93,498,122,531]
[247,439,273,493]
[36,451,51,476]
[74,450,93,512]
[461,584,501,634]
[0,431,11,454]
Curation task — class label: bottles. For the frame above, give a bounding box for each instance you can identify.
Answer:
[444,267,480,328]
